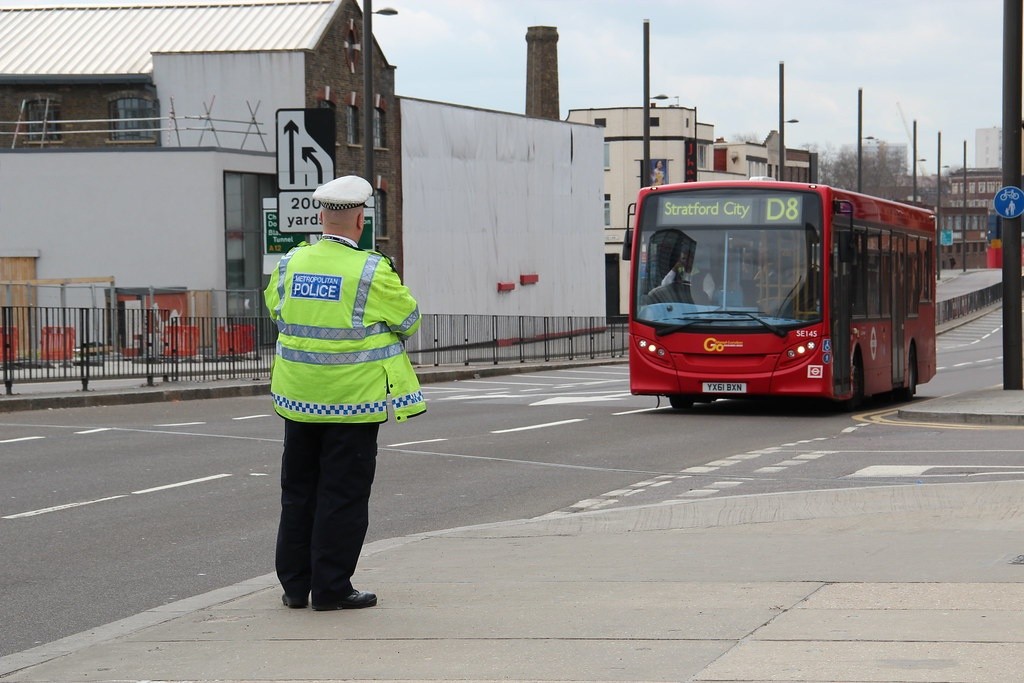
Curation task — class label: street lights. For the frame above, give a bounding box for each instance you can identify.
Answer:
[642,16,669,186]
[777,60,799,179]
[962,140,974,274]
[937,130,951,283]
[364,0,400,187]
[856,87,875,192]
[912,119,929,203]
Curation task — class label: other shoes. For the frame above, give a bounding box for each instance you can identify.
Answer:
[282,593,308,608]
[312,583,377,611]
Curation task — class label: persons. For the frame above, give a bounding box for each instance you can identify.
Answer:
[264,175,427,612]
[662,245,714,300]
[734,251,795,283]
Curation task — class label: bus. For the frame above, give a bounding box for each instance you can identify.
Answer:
[622,181,936,411]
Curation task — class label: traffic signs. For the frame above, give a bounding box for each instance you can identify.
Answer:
[276,106,341,237]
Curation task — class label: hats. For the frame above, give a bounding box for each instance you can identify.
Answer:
[312,175,373,210]
